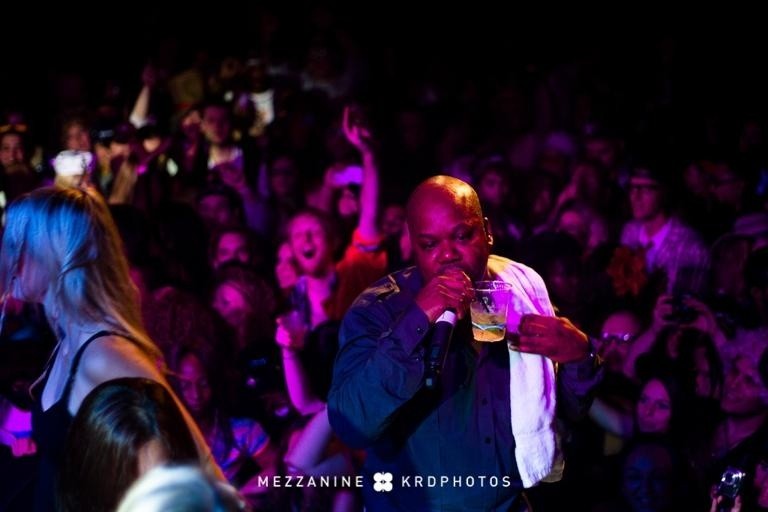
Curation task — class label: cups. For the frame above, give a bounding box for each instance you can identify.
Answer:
[466,281,513,342]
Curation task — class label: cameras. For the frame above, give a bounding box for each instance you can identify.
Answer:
[715,464,747,501]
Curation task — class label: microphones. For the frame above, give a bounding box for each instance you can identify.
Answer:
[428,265,464,370]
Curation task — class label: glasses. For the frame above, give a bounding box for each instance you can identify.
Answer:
[602,331,640,347]
